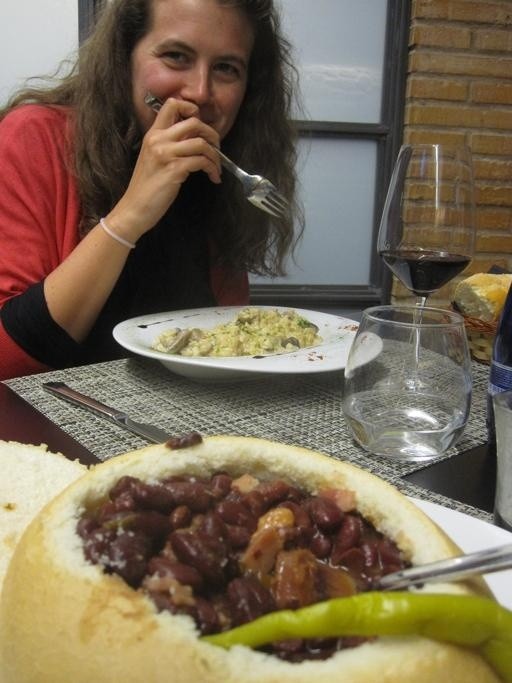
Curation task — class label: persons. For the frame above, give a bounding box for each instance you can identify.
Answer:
[2,0,313,395]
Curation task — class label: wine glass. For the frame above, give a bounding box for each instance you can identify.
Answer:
[376,141,477,391]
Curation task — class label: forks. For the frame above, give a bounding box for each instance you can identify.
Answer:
[141,89,291,219]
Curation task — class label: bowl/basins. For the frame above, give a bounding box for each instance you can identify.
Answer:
[110,302,385,383]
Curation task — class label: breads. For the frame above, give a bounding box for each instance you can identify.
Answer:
[0,433,503,683]
[454,273,512,322]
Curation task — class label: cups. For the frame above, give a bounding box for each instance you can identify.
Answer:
[487,390,512,531]
[339,303,477,463]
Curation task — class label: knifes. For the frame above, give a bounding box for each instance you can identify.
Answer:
[42,381,173,446]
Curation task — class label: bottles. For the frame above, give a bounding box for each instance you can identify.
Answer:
[484,282,511,481]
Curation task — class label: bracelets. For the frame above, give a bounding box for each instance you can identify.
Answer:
[99,217,138,249]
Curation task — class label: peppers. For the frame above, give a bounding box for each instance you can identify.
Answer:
[200,591,512,683]
